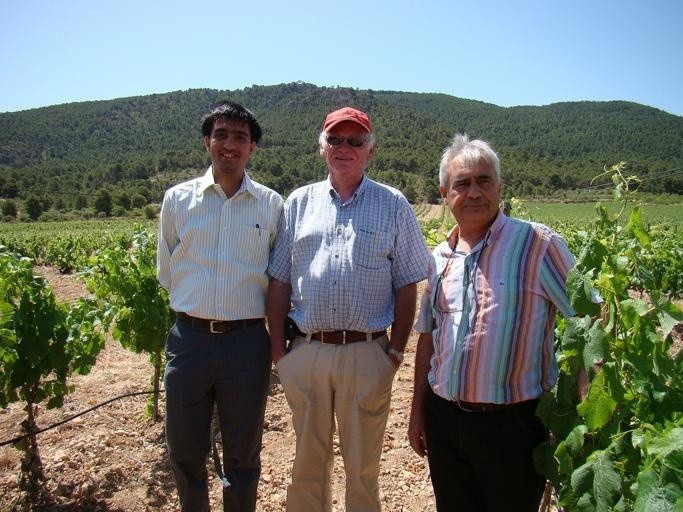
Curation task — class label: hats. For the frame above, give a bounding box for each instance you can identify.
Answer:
[323,106,372,135]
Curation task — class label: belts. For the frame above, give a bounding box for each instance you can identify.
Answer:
[292,329,387,346]
[174,311,264,335]
[455,399,516,415]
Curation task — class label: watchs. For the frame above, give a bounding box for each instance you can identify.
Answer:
[387,347,404,363]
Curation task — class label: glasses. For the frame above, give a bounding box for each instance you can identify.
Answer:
[324,135,369,147]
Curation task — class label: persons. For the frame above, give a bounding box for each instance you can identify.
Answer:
[408,130,603,512]
[264,106,430,512]
[156,99,283,512]
